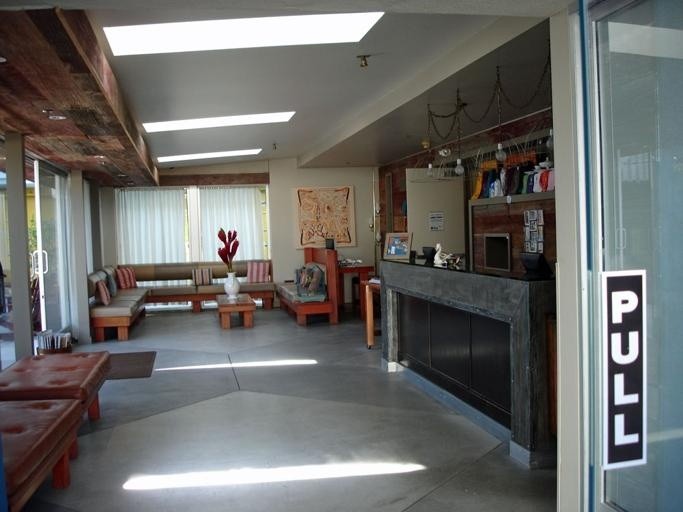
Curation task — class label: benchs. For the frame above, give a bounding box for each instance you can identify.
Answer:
[277,246,339,326]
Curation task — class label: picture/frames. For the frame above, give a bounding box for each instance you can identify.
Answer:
[428,210,446,231]
[383,232,413,262]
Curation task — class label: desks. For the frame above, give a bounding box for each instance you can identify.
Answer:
[359,278,381,350]
[338,263,373,314]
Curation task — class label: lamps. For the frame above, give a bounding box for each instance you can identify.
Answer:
[272,144,279,153]
[408,39,552,185]
[356,55,371,73]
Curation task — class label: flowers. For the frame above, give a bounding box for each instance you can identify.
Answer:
[217,228,239,273]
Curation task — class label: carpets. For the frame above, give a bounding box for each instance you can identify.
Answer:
[105,350,158,379]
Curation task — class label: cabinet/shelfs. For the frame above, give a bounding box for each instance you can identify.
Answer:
[395,290,511,432]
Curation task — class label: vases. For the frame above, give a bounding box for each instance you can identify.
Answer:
[223,272,241,299]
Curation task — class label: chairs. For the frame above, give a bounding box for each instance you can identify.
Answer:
[0,278,44,336]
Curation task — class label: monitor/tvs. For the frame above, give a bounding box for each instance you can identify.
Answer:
[520,252,553,277]
[423,246,436,263]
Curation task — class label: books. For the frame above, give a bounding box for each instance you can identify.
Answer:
[35,329,72,350]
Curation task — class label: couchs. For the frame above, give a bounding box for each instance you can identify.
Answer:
[0,350,110,422]
[87,259,276,341]
[0,398,83,511]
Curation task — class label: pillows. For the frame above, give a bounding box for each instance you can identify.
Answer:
[106,273,116,297]
[247,261,270,283]
[115,266,138,288]
[191,266,212,286]
[298,264,311,287]
[308,265,326,292]
[294,266,303,283]
[97,280,110,306]
